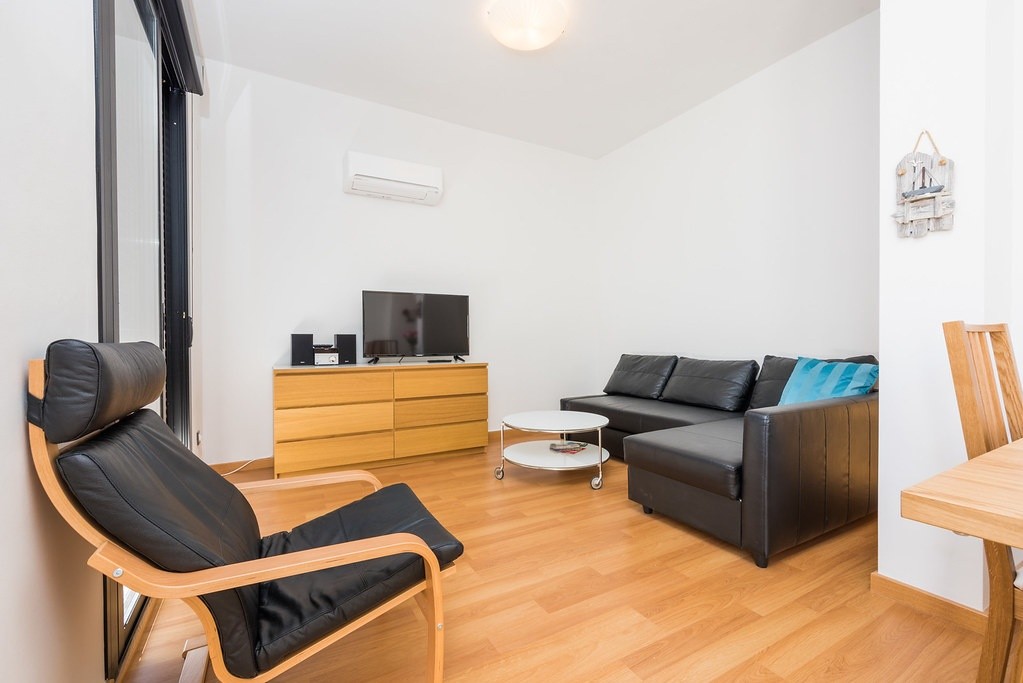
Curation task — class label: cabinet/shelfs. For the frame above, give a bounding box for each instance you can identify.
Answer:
[273,363,489,479]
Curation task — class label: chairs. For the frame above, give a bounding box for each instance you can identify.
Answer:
[28,338,465,683]
[942,321,1023,683]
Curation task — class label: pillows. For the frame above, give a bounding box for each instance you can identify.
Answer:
[777,356,878,405]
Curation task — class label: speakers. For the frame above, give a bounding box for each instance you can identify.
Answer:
[334,333,356,364]
[291,333,314,365]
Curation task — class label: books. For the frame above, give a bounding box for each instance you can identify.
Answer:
[550,443,581,450]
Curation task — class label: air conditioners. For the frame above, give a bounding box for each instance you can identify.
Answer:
[341,149,444,204]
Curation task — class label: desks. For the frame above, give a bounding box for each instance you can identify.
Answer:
[900,439,1023,550]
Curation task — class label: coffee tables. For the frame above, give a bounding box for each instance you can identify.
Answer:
[494,411,609,489]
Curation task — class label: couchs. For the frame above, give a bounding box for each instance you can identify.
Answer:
[559,353,879,570]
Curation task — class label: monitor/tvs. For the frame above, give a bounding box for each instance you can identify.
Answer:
[362,290,469,364]
